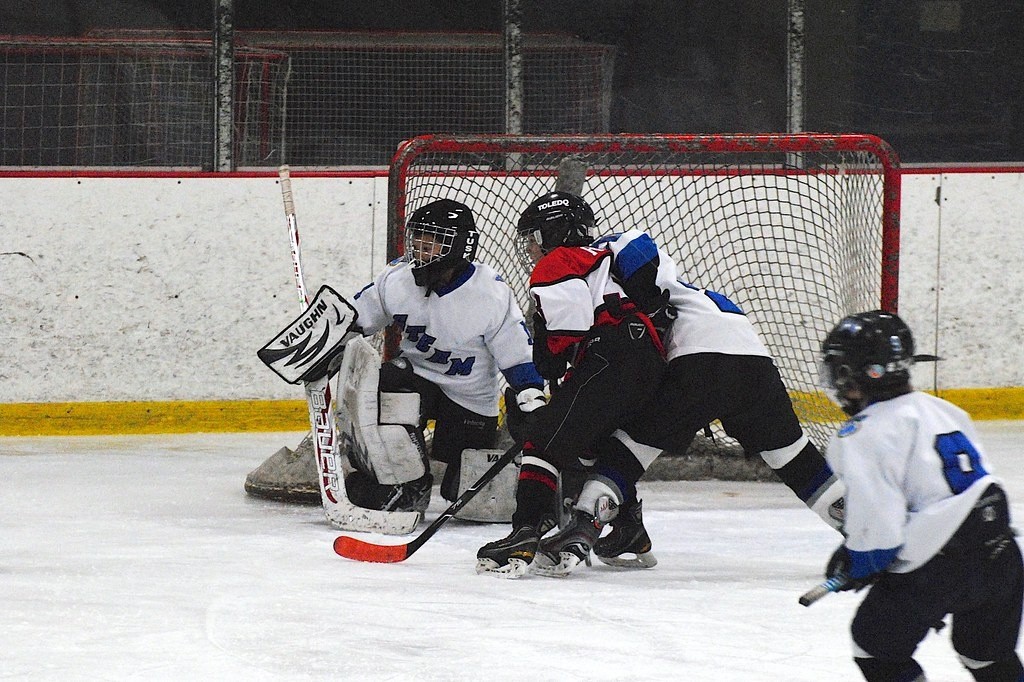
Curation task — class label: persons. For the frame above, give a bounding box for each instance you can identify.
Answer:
[304,199,547,523]
[821,310,1023,682]
[528,228,848,578]
[477,192,658,579]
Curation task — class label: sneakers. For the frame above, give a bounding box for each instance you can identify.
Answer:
[593,500,658,568]
[528,472,623,579]
[477,511,560,579]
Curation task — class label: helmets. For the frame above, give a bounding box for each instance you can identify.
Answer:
[818,310,916,416]
[402,200,480,287]
[513,192,595,277]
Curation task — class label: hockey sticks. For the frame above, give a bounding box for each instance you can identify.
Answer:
[332,441,520,563]
[280,167,421,536]
[798,569,855,608]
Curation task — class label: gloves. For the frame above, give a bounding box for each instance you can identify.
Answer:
[504,388,552,448]
[826,545,872,592]
